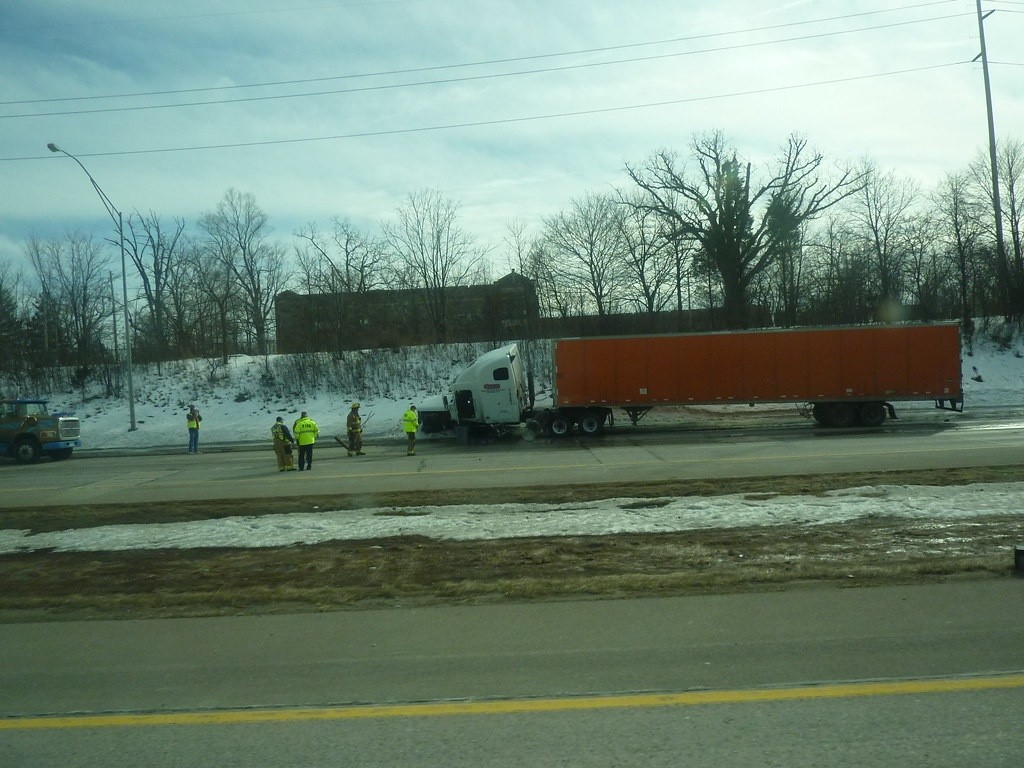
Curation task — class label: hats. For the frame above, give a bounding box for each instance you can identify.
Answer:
[301,412,307,416]
[276,417,284,421]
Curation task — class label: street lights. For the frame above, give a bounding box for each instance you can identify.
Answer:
[48,143,136,429]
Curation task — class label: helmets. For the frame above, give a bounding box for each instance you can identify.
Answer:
[350,403,360,408]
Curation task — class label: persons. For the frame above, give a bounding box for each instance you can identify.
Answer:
[270,416,295,472]
[293,411,318,471]
[347,403,366,457]
[186,405,202,455]
[402,406,419,456]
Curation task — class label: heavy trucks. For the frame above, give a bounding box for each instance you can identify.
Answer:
[441,320,964,470]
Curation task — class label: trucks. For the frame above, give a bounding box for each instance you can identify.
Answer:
[0,399,81,464]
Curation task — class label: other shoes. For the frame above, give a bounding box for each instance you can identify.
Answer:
[195,451,200,454]
[407,453,415,456]
[307,467,311,470]
[188,452,194,454]
[348,453,352,457]
[280,470,284,472]
[356,452,365,455]
[287,468,297,471]
[298,468,303,471]
